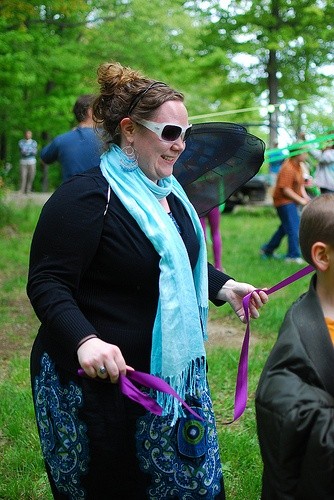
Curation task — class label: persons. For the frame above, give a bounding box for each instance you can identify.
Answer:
[255,193,334,500]
[261,145,312,264]
[40,95,106,183]
[17,129,38,195]
[298,130,334,194]
[26,59,268,500]
[199,206,224,272]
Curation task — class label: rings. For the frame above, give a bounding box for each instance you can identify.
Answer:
[97,367,106,375]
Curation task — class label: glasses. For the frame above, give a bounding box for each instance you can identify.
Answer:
[129,114,192,143]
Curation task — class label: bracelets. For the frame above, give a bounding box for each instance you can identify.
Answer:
[76,337,98,352]
